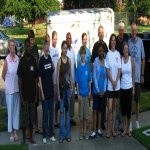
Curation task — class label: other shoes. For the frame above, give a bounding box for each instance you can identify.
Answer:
[105,135,110,139]
[128,132,132,137]
[68,139,71,142]
[121,131,127,137]
[89,132,97,138]
[112,132,117,137]
[70,119,76,127]
[97,131,103,137]
[43,138,46,144]
[79,135,88,140]
[27,135,30,140]
[60,140,63,143]
[10,135,19,142]
[49,136,56,141]
[30,141,36,144]
[35,128,42,134]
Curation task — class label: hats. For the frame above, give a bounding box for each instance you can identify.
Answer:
[28,30,35,38]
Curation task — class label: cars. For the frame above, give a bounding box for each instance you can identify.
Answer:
[2,17,16,28]
[0,30,23,60]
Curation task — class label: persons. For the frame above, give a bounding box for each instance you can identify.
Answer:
[1,20,146,145]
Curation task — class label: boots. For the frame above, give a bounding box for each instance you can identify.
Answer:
[135,121,140,128]
[129,122,133,132]
